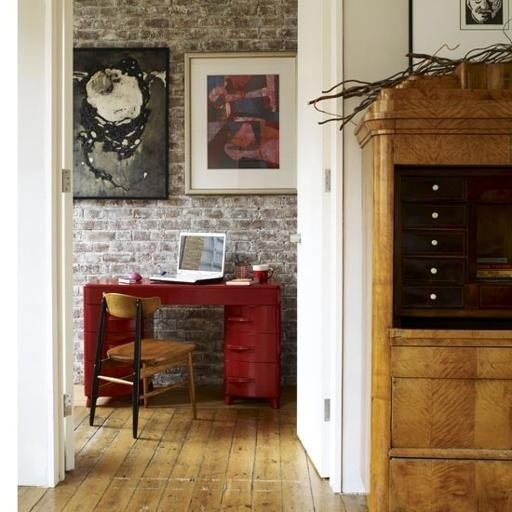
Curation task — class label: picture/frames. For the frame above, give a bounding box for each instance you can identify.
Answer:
[182,47,298,200]
[407,0,512,77]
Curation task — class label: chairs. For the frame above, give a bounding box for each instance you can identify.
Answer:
[89,291,197,438]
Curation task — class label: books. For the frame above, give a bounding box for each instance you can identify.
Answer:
[476,263,511,270]
[233,278,251,282]
[477,256,508,263]
[475,269,511,279]
[225,279,255,286]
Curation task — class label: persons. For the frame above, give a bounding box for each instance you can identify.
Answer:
[464,0,503,24]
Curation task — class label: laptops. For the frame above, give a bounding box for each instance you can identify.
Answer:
[149,232,227,284]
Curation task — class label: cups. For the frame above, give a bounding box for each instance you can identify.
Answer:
[235,262,250,279]
[252,264,274,284]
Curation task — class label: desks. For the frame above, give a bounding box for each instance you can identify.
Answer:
[84,277,282,409]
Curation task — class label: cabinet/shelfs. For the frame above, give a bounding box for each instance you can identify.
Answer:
[353,60,512,511]
